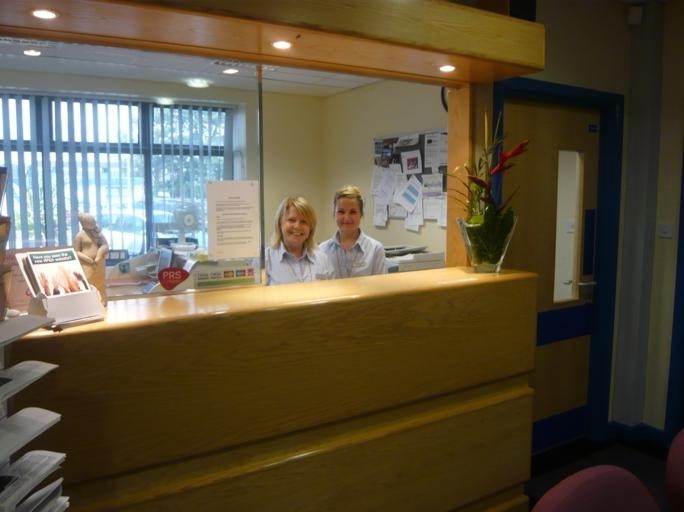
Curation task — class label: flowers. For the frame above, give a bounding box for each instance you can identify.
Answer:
[442,111,530,262]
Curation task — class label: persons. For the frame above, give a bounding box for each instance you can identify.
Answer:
[318,184,386,280]
[74,211,110,308]
[262,194,330,285]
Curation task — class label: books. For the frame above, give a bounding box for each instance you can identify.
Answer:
[0,265,12,323]
[0,165,8,213]
[14,247,89,297]
[0,215,11,268]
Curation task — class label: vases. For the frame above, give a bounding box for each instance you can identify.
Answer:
[455,218,519,273]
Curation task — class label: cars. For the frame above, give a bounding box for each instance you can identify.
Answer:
[92,193,208,258]
